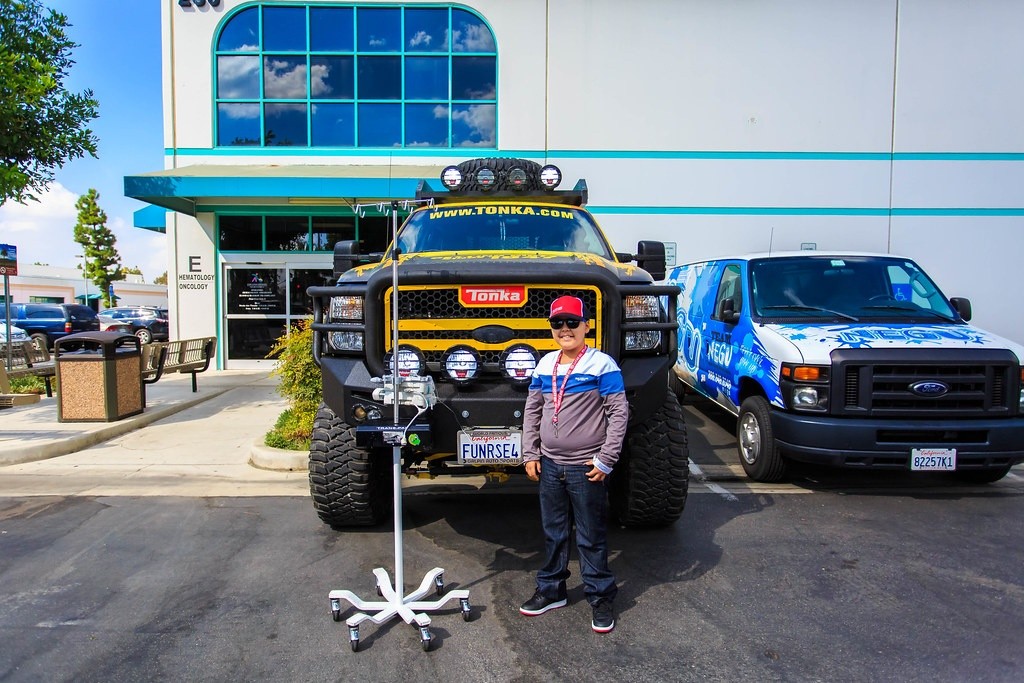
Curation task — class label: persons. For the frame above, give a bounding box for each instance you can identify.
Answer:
[518,295,628,633]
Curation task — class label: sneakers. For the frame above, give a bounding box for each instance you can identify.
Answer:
[520,587,568,616]
[591,593,615,633]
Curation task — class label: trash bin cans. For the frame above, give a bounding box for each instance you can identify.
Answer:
[54,331,145,423]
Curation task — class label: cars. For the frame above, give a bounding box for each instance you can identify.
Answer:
[96,314,133,346]
[0,321,33,356]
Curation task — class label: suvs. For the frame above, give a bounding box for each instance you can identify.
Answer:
[97,306,170,346]
[305,161,688,526]
[658,250,1023,483]
[0,302,98,352]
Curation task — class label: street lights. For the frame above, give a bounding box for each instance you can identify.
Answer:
[75,253,89,307]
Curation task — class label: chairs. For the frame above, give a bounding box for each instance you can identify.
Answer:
[827,267,870,314]
[723,271,778,312]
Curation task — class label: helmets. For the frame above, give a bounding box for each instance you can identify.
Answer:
[547,295,587,322]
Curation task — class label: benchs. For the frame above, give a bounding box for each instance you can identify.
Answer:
[0,339,55,398]
[141,337,218,408]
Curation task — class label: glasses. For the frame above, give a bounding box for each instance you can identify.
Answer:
[550,318,584,330]
